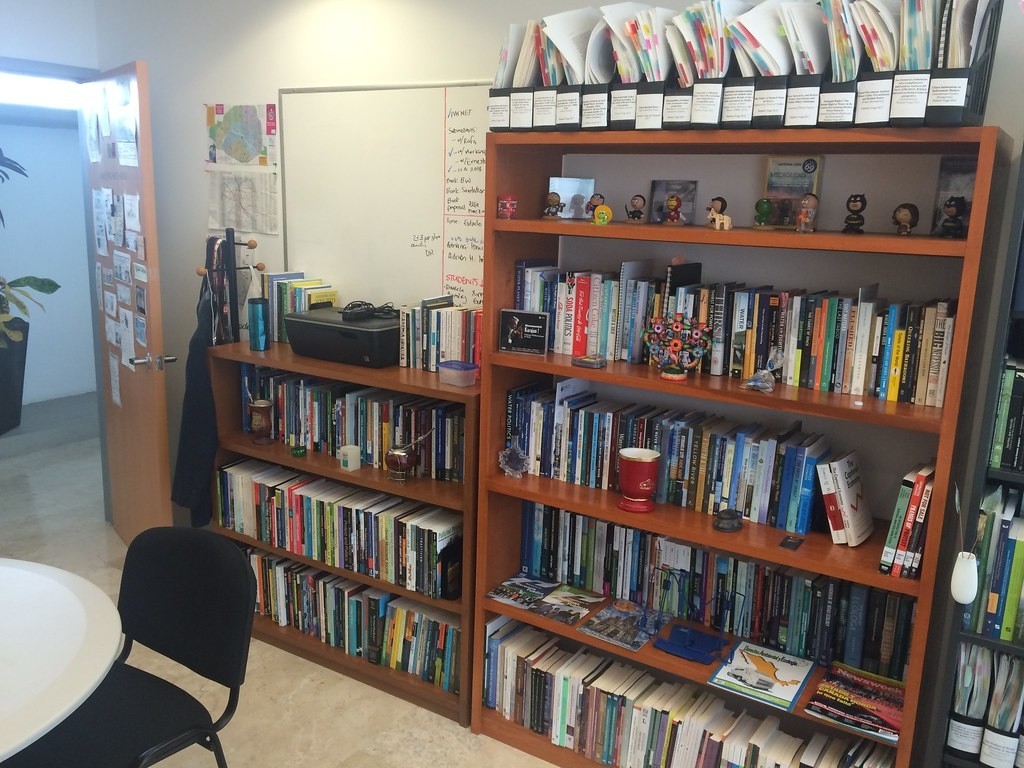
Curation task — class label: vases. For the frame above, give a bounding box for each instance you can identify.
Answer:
[617,448,660,513]
[249,399,274,444]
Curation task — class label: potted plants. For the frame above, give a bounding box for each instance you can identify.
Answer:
[0,148,61,435]
[951,483,1003,603]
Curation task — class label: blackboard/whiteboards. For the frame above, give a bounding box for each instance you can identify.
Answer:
[277,78,493,310]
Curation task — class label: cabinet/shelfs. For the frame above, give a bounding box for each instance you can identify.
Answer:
[920,141,1024,768]
[206,341,482,727]
[468,124,1015,768]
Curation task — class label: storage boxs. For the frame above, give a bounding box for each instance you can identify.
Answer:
[436,360,479,387]
[340,445,360,472]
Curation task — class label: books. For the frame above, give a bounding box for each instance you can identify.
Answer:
[423,604,462,694]
[259,270,338,346]
[925,301,949,406]
[763,155,825,230]
[334,491,394,583]
[240,551,284,628]
[337,387,468,485]
[237,360,336,461]
[945,640,1023,768]
[335,584,381,665]
[381,596,422,677]
[284,568,334,648]
[221,464,284,550]
[956,362,1024,638]
[481,255,937,768]
[285,474,334,567]
[935,315,955,409]
[397,293,484,382]
[394,486,462,592]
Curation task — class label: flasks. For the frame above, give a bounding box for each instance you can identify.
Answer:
[247,298,270,351]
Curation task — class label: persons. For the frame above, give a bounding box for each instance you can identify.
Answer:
[542,191,966,237]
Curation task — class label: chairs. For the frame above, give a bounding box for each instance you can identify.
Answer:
[0,526,257,768]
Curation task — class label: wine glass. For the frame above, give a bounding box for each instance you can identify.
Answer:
[248,399,274,445]
[617,448,662,512]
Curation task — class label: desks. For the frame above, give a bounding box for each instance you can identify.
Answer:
[0,557,123,764]
